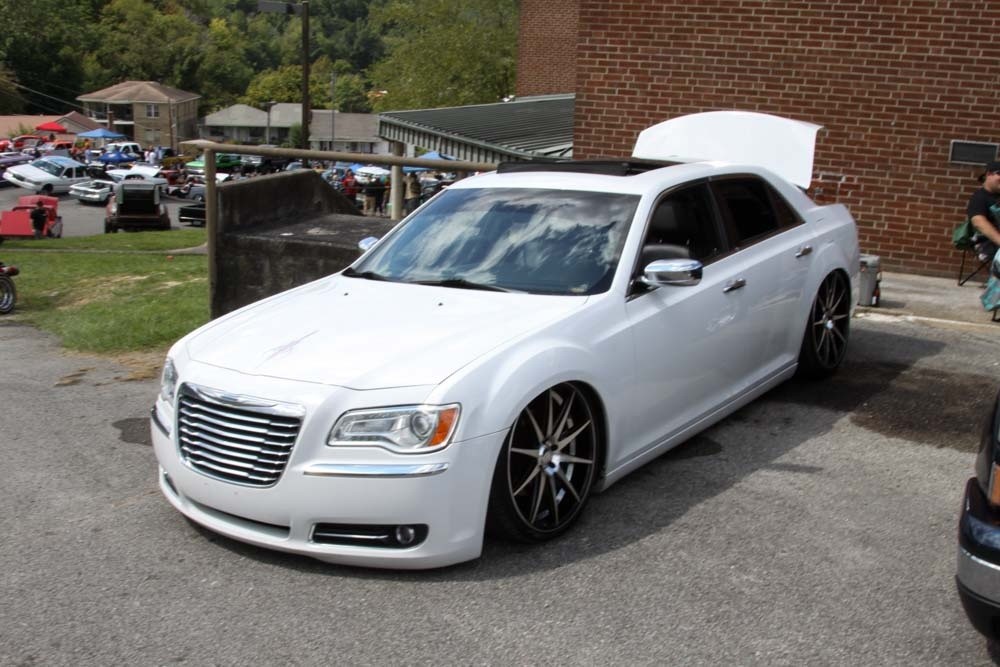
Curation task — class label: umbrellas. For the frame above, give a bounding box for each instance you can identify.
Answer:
[357,167,389,174]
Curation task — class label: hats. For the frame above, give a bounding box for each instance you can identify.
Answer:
[977,162,1000,181]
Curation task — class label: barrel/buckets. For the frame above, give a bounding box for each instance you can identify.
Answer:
[858,254,881,308]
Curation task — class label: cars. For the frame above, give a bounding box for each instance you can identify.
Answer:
[2,155,91,196]
[0,140,455,238]
[147,109,861,572]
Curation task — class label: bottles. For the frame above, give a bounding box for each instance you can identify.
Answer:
[871,279,881,308]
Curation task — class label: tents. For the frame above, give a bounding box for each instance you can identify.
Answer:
[72,128,128,149]
[403,152,452,171]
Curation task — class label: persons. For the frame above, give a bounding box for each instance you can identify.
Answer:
[222,172,257,182]
[145,145,164,164]
[70,140,93,164]
[315,165,391,216]
[403,172,419,214]
[30,201,46,239]
[967,161,1000,270]
[31,147,41,158]
[8,139,15,152]
